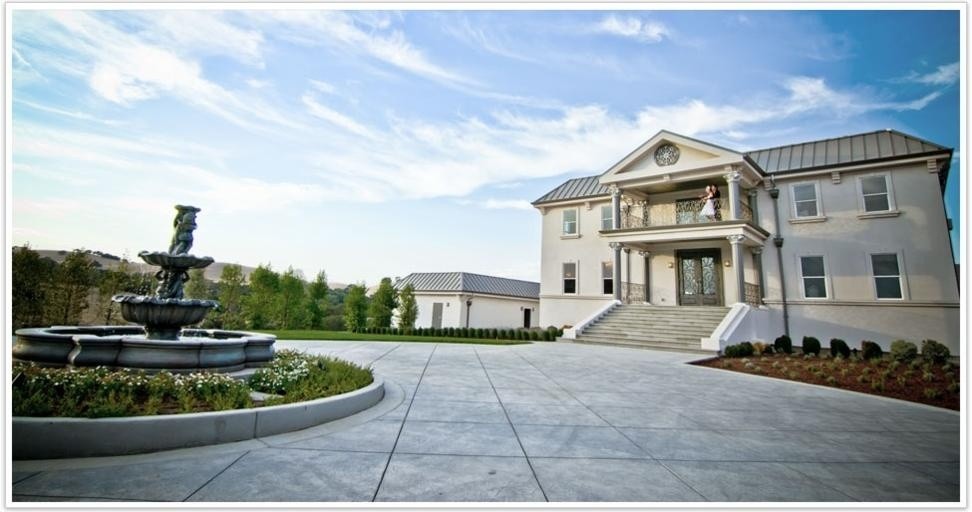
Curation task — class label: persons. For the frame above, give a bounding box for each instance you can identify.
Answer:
[175,212,198,256]
[174,270,191,297]
[153,269,165,296]
[698,184,716,222]
[703,182,723,222]
